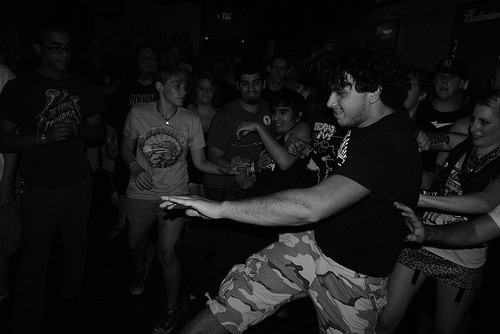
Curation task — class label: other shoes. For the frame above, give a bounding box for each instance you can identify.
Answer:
[0,291,9,301]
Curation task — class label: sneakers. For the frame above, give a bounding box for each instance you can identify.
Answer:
[129,276,146,294]
[153,304,181,334]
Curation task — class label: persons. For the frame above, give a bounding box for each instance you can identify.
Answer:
[0,61,16,301]
[374,98,500,334]
[393,201,500,250]
[205,62,280,294]
[402,67,436,137]
[80,33,126,112]
[261,55,291,97]
[124,69,251,334]
[103,42,165,221]
[158,29,420,334]
[235,87,313,196]
[181,71,221,233]
[0,21,109,334]
[418,54,477,186]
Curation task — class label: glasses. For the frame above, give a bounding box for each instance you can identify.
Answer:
[46,46,72,54]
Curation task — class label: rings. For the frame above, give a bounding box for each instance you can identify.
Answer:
[237,129,240,132]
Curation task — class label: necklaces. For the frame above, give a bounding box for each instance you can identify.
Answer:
[157,104,178,126]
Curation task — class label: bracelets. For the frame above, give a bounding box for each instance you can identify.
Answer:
[129,160,137,170]
[40,132,47,144]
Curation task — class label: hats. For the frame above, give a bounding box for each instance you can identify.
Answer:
[434,52,467,79]
